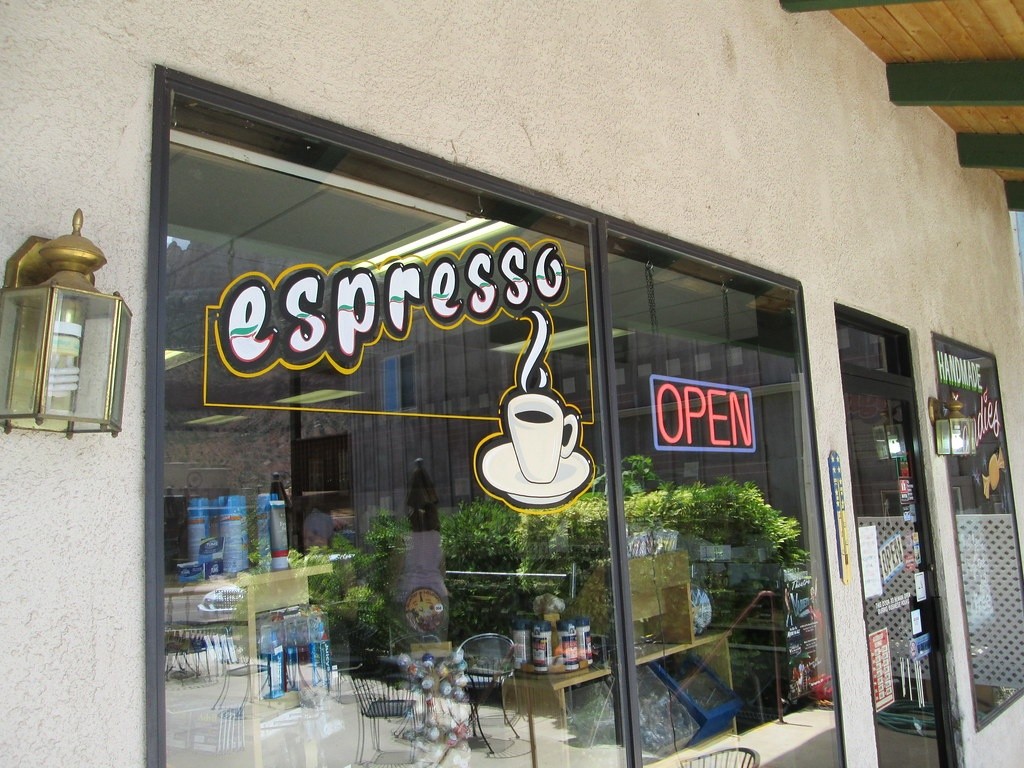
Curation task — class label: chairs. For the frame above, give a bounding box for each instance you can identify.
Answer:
[679,748,761,768]
[451,633,521,738]
[335,663,419,765]
[161,598,363,720]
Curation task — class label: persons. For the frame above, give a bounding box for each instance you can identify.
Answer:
[388,504,449,642]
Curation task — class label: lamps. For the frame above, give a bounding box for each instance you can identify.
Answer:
[1,208,133,439]
[872,405,905,460]
[928,389,976,457]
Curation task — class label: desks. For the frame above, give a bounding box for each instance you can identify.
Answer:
[457,667,521,754]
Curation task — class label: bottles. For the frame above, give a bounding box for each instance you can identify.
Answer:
[188,495,248,571]
[556,620,579,670]
[531,621,552,671]
[576,618,593,665]
[511,619,530,669]
[256,493,278,565]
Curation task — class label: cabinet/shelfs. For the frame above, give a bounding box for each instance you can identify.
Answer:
[164,564,333,768]
[505,550,738,768]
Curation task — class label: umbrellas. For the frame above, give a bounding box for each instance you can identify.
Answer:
[264,474,294,571]
[406,458,442,503]
